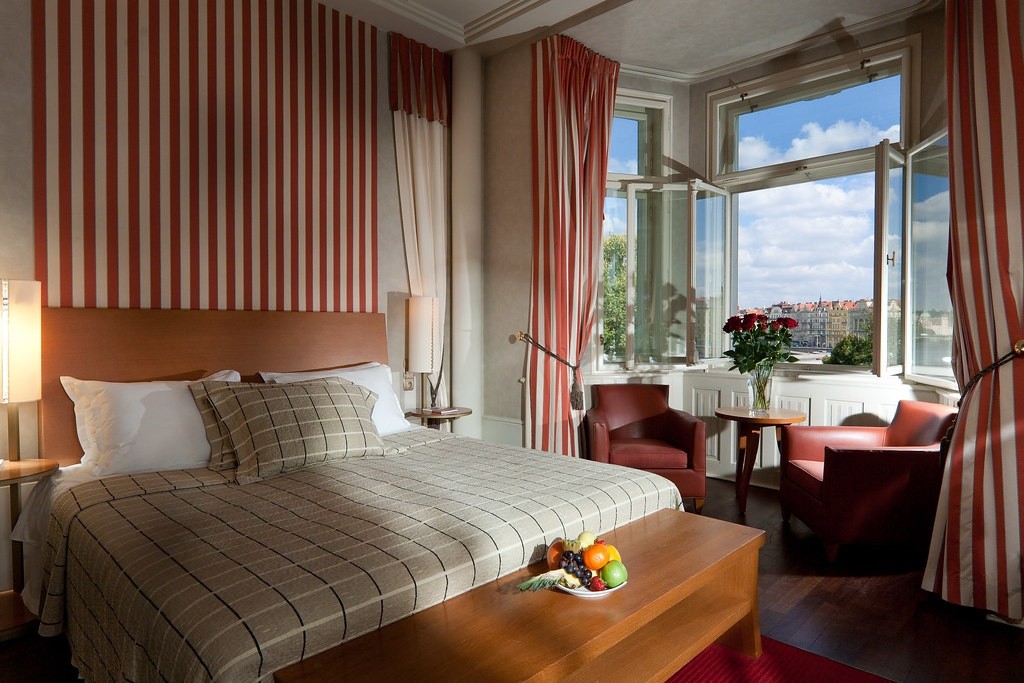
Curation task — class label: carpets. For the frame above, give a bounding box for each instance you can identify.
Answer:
[665,635,899,683]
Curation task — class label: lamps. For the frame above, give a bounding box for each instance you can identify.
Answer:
[409,298,439,427]
[1,280,42,462]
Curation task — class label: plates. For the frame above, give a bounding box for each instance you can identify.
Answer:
[554,581,627,599]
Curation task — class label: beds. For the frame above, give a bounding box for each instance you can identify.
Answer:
[32,305,685,683]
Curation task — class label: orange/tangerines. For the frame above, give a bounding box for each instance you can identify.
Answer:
[581,544,610,570]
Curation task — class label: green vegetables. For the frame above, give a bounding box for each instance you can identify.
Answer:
[518,568,580,592]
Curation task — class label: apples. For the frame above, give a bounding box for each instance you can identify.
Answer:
[547,540,565,570]
[599,560,628,588]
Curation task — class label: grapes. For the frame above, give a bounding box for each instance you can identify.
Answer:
[560,550,592,586]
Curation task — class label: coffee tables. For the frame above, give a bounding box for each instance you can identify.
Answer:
[714,408,807,513]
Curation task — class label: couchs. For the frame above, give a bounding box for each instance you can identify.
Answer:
[778,401,961,564]
[585,384,707,516]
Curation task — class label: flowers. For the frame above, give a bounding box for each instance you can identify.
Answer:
[722,313,799,408]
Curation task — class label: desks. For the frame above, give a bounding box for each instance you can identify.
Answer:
[273,509,765,683]
[0,459,60,643]
[412,405,472,432]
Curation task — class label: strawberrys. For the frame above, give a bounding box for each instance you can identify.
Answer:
[589,576,607,591]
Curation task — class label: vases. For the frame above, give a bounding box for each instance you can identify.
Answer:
[748,363,773,413]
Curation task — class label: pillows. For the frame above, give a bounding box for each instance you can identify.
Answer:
[189,377,253,471]
[59,370,241,478]
[205,382,384,484]
[260,360,412,436]
